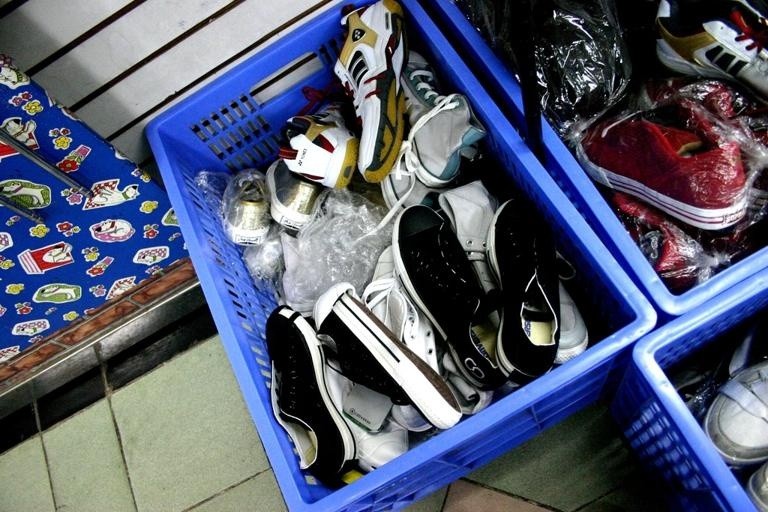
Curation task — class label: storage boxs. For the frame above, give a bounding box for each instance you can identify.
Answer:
[610,268,768,512]
[143,0,660,512]
[435,0,768,317]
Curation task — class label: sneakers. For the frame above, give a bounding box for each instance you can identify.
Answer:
[223,0,592,485]
[746,462,768,512]
[703,360,767,465]
[527,0,767,281]
